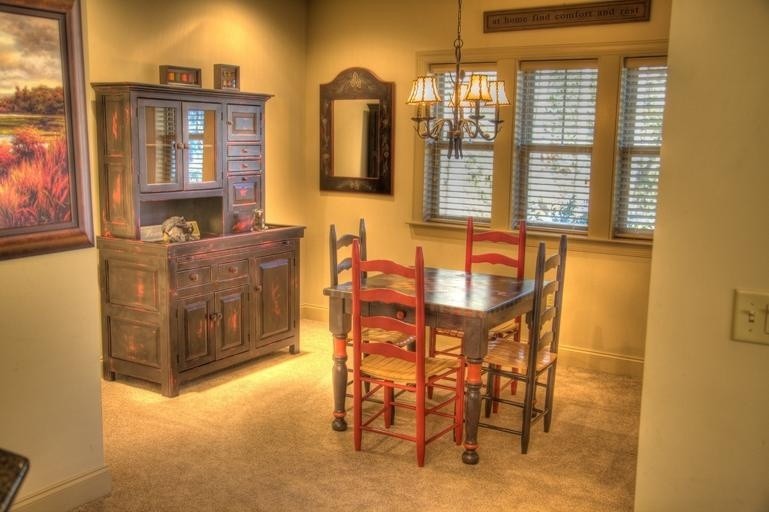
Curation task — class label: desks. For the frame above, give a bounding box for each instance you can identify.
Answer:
[323,265,549,463]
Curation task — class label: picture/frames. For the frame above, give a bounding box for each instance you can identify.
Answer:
[0,0,94,260]
[319,67,395,195]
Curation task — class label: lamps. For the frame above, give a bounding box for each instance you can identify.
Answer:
[403,0,511,159]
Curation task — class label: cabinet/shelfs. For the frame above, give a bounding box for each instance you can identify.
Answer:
[91,81,306,398]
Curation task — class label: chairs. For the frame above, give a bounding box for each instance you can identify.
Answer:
[452,233,566,454]
[328,218,420,431]
[424,218,527,416]
[351,237,466,467]
